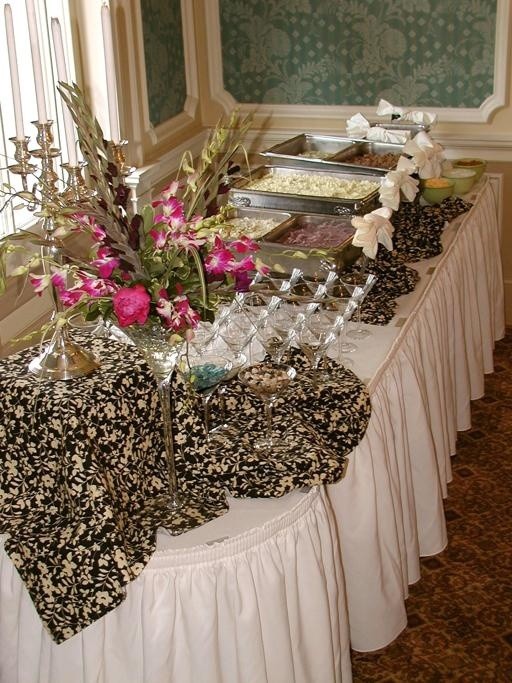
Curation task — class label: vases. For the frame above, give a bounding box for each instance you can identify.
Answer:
[115,320,205,509]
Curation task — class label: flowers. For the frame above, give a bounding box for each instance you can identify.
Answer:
[0,80,289,386]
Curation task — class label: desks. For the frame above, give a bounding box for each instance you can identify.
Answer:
[0,173,504,683]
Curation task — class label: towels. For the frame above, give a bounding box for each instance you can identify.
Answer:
[346,97,446,259]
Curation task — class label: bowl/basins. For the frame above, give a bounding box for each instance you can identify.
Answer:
[420,158,488,205]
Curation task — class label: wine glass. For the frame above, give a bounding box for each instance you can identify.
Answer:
[179,268,378,455]
[105,317,198,510]
